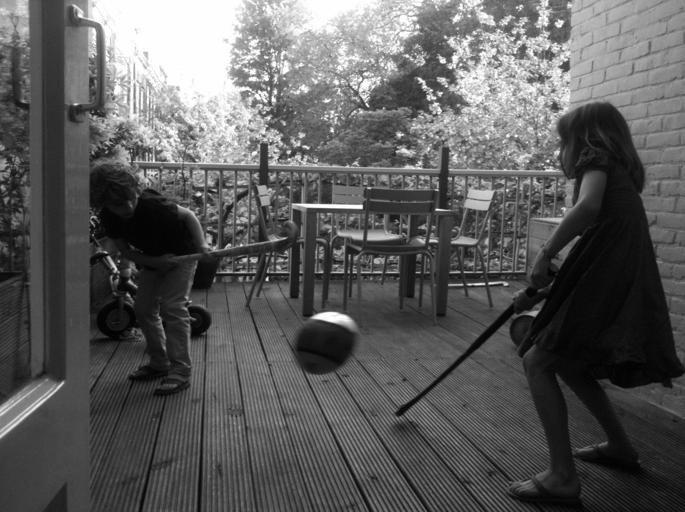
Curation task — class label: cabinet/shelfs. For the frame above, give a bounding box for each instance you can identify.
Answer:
[524,217,582,290]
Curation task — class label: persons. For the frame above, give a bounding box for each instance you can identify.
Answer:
[88,161,218,397]
[506,101,685,504]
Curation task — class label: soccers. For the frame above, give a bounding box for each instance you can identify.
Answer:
[294,310,359,375]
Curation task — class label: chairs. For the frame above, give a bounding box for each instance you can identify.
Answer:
[245,183,496,326]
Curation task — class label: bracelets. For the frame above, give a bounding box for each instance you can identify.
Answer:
[539,241,563,262]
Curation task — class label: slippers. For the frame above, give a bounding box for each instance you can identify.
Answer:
[154,377,191,397]
[505,476,581,505]
[127,364,165,381]
[574,442,641,469]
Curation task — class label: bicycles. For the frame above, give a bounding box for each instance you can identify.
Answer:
[89,212,212,340]
